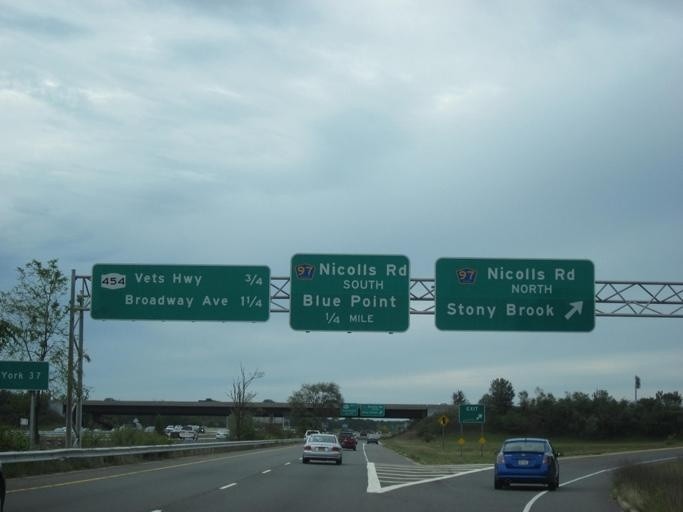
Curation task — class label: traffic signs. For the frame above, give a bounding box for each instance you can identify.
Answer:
[432,256,597,334]
[339,402,359,417]
[358,403,386,418]
[0,359,51,391]
[458,403,486,425]
[287,251,412,334]
[89,262,272,324]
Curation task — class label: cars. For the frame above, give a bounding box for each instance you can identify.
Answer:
[198,397,220,402]
[492,436,562,492]
[103,397,115,401]
[302,433,343,466]
[215,428,231,440]
[48,422,206,443]
[0,459,7,512]
[302,427,392,451]
[262,399,274,402]
